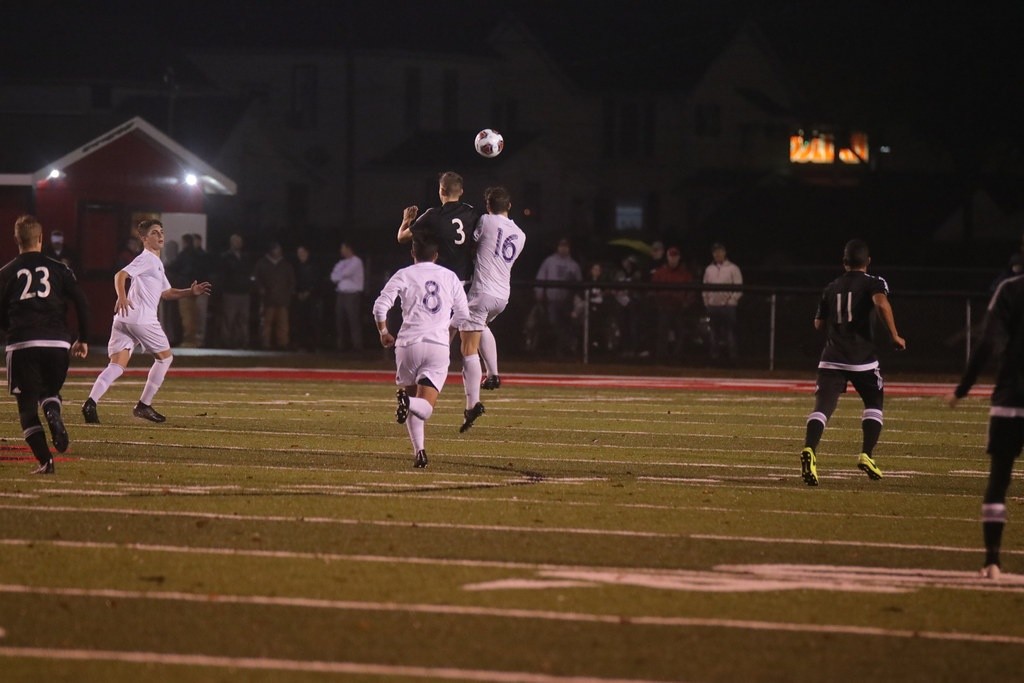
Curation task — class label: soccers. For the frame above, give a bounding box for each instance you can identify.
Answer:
[474,128,505,158]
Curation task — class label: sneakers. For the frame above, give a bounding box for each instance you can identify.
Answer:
[44,401,69,452]
[481,375,500,390]
[857,451,883,481]
[33,458,55,474]
[394,388,410,424]
[81,398,100,424]
[800,446,819,486]
[459,402,485,433]
[412,450,427,469]
[133,401,167,423]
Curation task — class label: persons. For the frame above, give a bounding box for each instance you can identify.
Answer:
[47,231,75,269]
[801,240,906,486]
[171,233,209,347]
[83,220,211,423]
[397,172,479,345]
[255,241,328,350]
[702,243,743,361]
[373,235,469,468]
[216,235,255,348]
[458,187,526,433]
[331,242,364,349]
[951,247,1024,583]
[0,215,89,475]
[535,239,699,362]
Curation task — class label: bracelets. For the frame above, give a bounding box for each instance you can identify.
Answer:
[379,328,388,336]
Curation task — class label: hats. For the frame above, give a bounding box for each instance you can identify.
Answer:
[558,238,572,247]
[665,247,680,258]
[711,242,725,252]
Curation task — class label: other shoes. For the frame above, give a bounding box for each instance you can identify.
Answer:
[980,565,1002,582]
